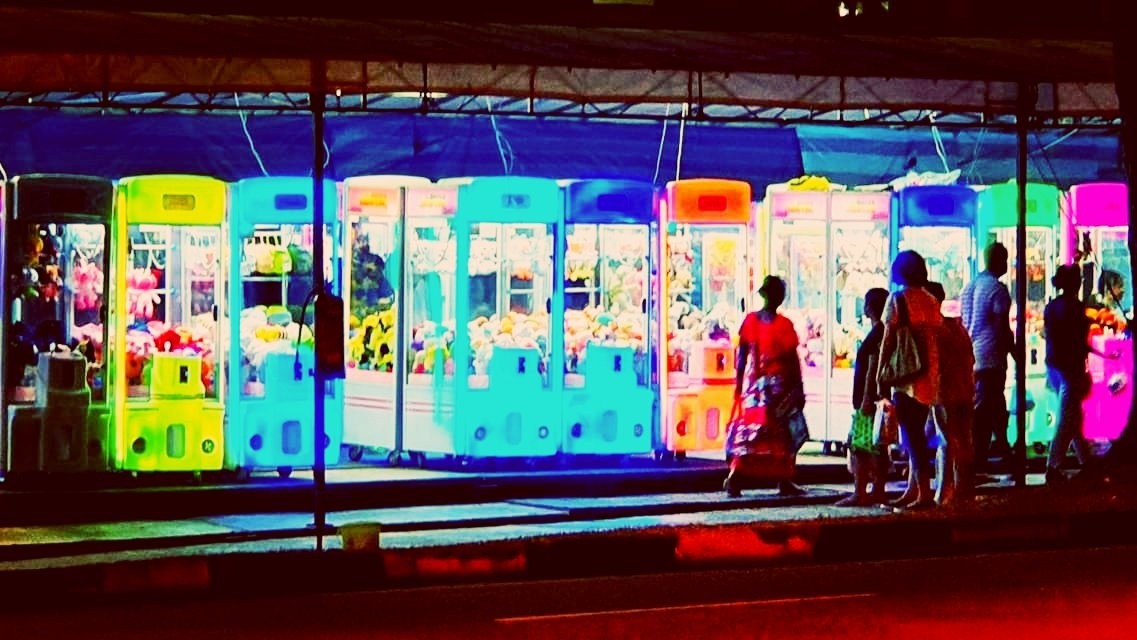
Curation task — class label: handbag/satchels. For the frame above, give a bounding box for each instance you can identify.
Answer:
[878,293,923,380]
[849,410,881,457]
[872,398,900,449]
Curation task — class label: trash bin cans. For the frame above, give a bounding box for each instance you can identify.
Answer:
[37,351,89,472]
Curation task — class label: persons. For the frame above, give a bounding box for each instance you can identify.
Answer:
[1041,264,1101,483]
[1095,270,1129,326]
[960,242,1030,481]
[723,274,809,496]
[834,250,975,509]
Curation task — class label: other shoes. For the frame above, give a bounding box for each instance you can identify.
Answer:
[834,458,1103,512]
[723,479,744,498]
[779,483,809,496]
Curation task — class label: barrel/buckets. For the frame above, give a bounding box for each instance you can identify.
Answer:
[337,519,381,551]
